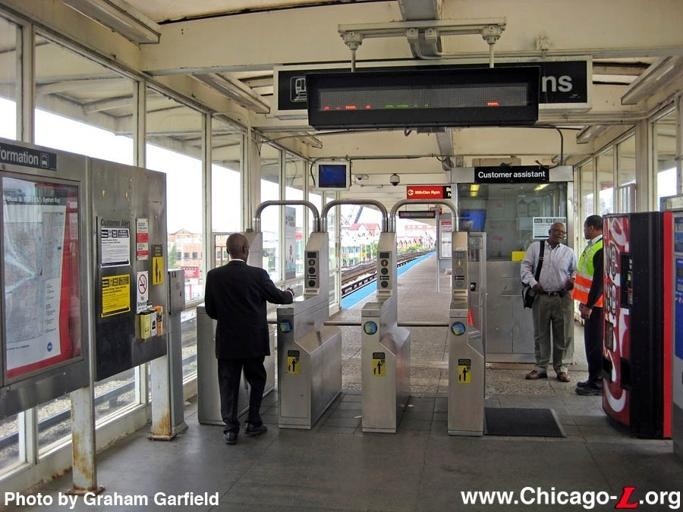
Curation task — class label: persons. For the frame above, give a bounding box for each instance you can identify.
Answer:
[521,222,577,383]
[571,215,604,396]
[205,233,294,445]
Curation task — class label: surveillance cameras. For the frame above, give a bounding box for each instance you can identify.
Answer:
[390,175,400,187]
[357,175,362,180]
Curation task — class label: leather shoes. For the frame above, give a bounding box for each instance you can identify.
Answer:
[575,386,602,396]
[576,380,589,386]
[526,369,547,379]
[557,371,570,382]
[244,423,267,436]
[223,431,237,444]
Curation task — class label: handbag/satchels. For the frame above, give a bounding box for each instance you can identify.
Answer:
[521,240,545,309]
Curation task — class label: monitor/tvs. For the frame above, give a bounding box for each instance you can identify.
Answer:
[316,161,350,193]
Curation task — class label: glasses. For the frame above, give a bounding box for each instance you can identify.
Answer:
[550,228,565,234]
